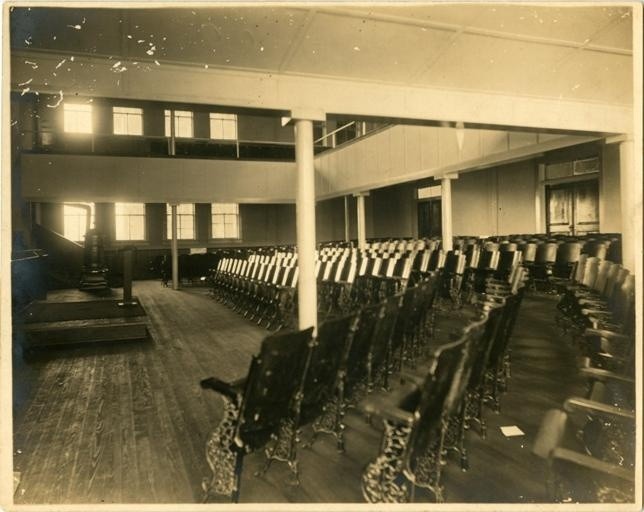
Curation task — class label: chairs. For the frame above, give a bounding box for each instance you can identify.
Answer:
[157,231,634,504]
